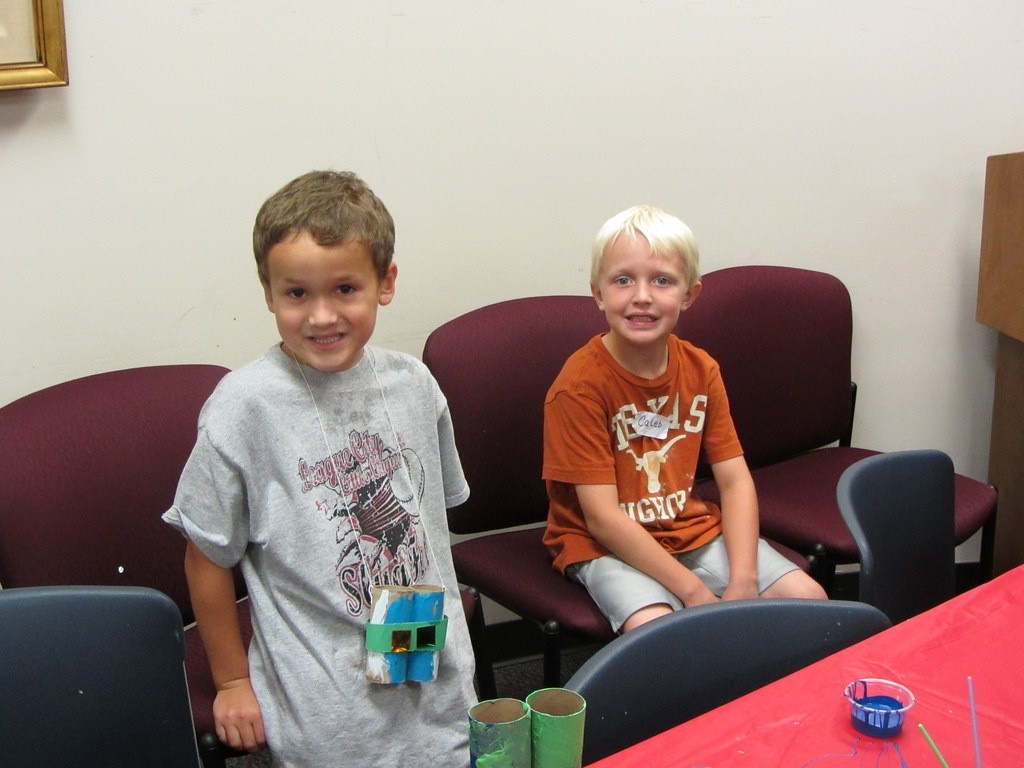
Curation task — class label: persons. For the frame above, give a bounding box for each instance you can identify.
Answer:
[543,202,829,635]
[163,171,479,768]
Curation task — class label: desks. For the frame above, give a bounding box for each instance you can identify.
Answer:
[584,564,1024,768]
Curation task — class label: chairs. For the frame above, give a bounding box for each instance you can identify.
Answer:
[834,449,958,622]
[0,364,267,768]
[421,295,812,699]
[680,265,998,601]
[563,597,892,768]
[0,584,202,768]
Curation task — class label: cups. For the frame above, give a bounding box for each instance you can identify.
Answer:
[844,678,915,737]
